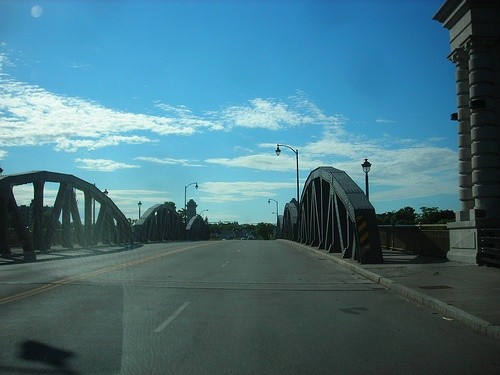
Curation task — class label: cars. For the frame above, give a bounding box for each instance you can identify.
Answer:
[240,235,257,240]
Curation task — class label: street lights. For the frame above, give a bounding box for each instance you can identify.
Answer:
[275,143,299,203]
[361,158,372,199]
[185,182,199,218]
[268,198,278,239]
[138,201,142,218]
